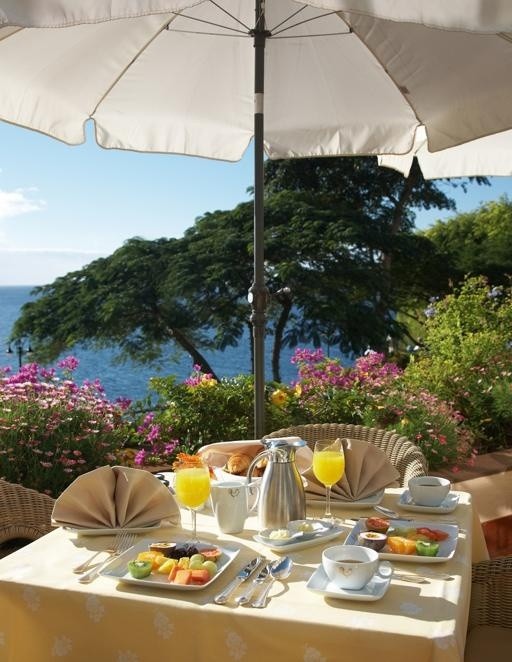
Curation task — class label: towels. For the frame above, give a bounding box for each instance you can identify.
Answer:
[294,438,400,503]
[51,465,181,529]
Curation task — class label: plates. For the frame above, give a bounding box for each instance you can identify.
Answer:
[98,537,241,590]
[343,518,458,563]
[252,517,344,554]
[60,521,162,534]
[307,561,394,601]
[396,490,460,514]
[306,488,385,509]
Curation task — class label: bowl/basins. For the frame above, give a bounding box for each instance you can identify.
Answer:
[408,477,451,506]
[322,546,381,590]
[286,519,325,537]
[260,527,303,546]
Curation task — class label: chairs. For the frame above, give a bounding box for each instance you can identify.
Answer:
[0,479,57,560]
[465,557,512,662]
[263,423,429,488]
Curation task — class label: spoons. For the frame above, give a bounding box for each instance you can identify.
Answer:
[374,504,400,520]
[252,556,292,609]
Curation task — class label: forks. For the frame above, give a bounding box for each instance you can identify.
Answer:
[75,530,128,573]
[79,533,138,584]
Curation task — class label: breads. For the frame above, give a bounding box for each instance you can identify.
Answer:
[227,447,268,478]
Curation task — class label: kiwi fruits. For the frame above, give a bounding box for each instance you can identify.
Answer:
[127,560,152,578]
[416,541,440,556]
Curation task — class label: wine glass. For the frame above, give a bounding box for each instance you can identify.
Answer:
[313,441,345,520]
[176,463,211,544]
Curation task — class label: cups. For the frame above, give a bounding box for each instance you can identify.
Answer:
[209,482,262,535]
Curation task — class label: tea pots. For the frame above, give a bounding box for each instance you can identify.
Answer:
[246,437,307,528]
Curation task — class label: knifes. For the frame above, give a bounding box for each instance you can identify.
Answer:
[215,555,263,606]
[236,561,276,605]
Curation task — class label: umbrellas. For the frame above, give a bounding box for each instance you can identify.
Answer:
[1,0,511,438]
